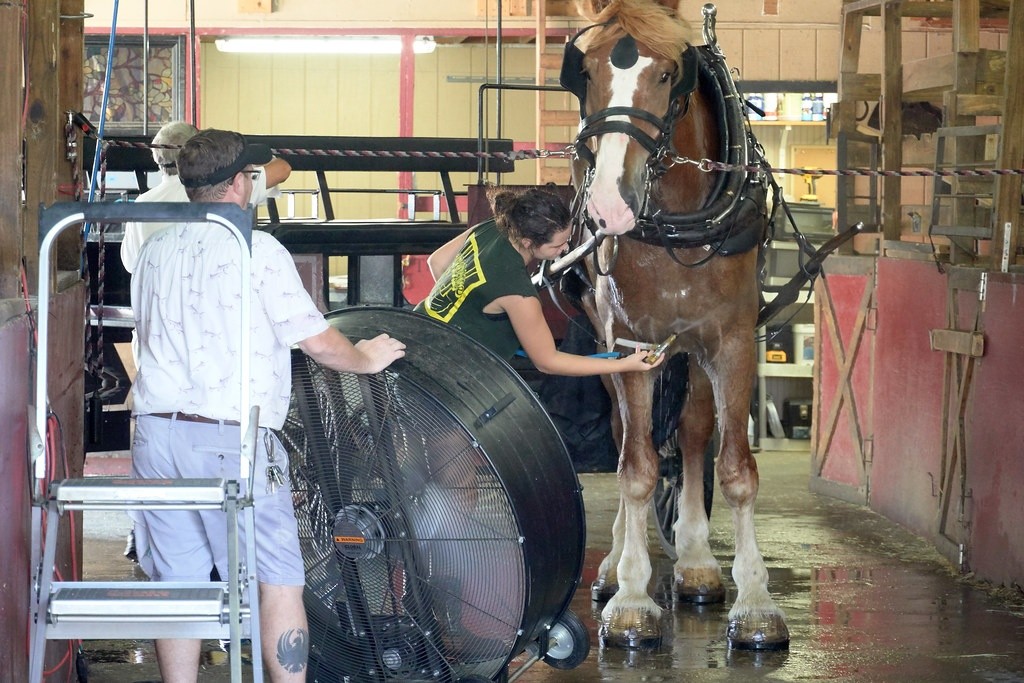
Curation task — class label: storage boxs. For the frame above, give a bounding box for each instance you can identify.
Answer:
[767,323,817,366]
[772,202,835,243]
[787,398,812,438]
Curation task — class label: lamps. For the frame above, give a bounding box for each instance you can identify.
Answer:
[214,35,436,54]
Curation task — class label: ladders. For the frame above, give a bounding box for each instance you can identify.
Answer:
[28,199,265,683]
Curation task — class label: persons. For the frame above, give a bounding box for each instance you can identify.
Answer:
[383,187,666,665]
[120,120,407,683]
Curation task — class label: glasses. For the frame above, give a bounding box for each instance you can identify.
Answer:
[227,170,261,185]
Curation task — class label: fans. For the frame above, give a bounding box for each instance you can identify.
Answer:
[284,305,587,683]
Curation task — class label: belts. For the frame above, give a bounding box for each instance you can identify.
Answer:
[147,411,241,426]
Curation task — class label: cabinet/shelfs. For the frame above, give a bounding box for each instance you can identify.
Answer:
[746,119,840,451]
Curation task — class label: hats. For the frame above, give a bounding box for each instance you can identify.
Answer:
[179,132,272,189]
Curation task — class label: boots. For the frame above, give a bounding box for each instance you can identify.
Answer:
[428,575,508,659]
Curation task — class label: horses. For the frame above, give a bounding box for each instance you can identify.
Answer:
[563,0,791,650]
